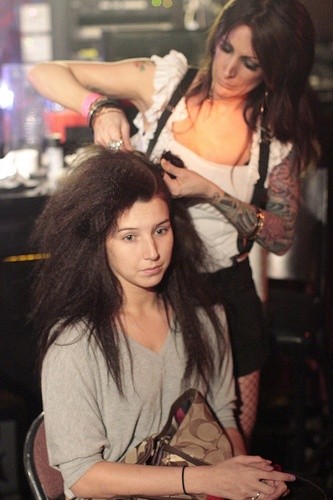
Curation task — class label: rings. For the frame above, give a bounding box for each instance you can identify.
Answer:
[251,491,260,500]
[108,139,124,153]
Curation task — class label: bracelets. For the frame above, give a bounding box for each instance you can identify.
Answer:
[245,205,265,241]
[182,465,188,496]
[80,93,124,133]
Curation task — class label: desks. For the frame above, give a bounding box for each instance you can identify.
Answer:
[0,175,52,411]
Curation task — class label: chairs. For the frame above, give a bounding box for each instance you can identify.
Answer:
[21,411,64,500]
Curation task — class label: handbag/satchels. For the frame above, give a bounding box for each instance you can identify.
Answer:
[70,388,235,500]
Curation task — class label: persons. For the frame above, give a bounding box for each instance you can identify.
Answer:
[30,0,316,453]
[26,142,297,500]
